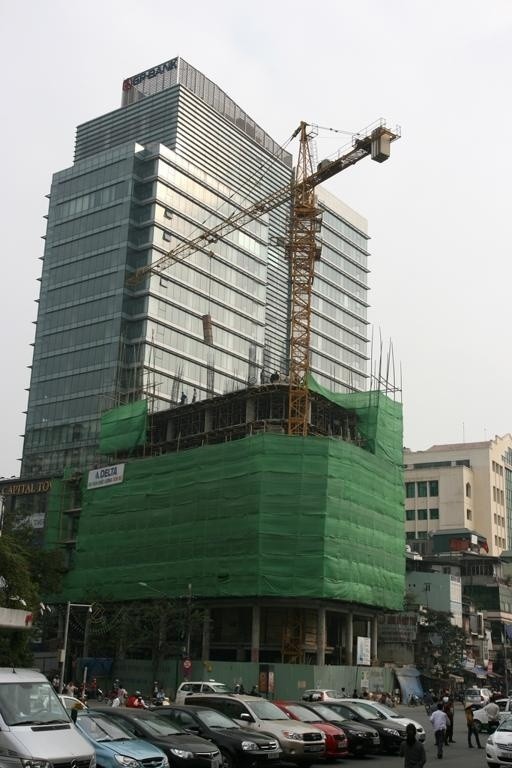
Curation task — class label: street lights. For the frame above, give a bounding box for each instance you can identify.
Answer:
[138,582,192,682]
[59,603,93,695]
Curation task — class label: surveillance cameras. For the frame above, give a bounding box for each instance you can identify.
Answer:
[88,607,93,613]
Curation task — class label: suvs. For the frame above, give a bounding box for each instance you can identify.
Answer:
[182,692,327,768]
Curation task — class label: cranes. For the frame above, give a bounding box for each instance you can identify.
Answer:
[127,117,402,444]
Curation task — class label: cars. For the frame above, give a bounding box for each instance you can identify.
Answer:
[86,705,229,768]
[272,700,349,765]
[146,705,284,768]
[30,694,88,712]
[296,701,381,760]
[318,700,419,756]
[335,698,426,744]
[66,709,170,768]
[463,688,512,768]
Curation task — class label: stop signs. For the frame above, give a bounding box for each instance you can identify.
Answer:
[183,658,193,669]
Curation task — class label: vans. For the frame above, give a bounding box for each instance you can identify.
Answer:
[300,689,351,702]
[0,666,97,768]
[174,681,242,705]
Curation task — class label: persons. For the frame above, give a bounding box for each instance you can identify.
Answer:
[52,672,166,707]
[464,700,483,749]
[429,703,451,758]
[339,681,463,707]
[398,722,427,767]
[486,696,502,733]
[443,703,456,746]
